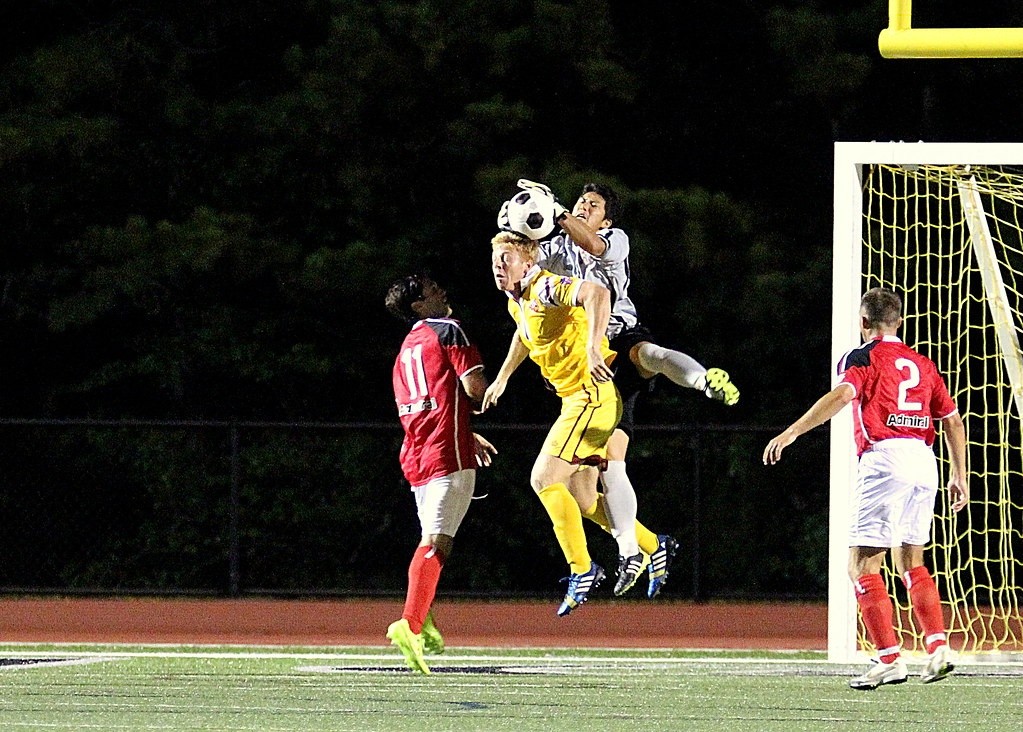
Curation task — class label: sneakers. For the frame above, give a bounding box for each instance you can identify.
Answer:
[653,535,680,598]
[420,610,446,655]
[852,656,908,691]
[926,647,956,683]
[613,552,660,593]
[704,368,740,406]
[386,618,431,676]
[557,563,606,618]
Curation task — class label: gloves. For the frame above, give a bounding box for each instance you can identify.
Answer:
[497,200,512,230]
[517,176,568,223]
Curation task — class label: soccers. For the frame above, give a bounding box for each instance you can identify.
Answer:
[507,190,556,240]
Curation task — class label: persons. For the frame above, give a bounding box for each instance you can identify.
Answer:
[481,233,679,616]
[498,179,740,596]
[763,288,969,691]
[386,275,498,676]
